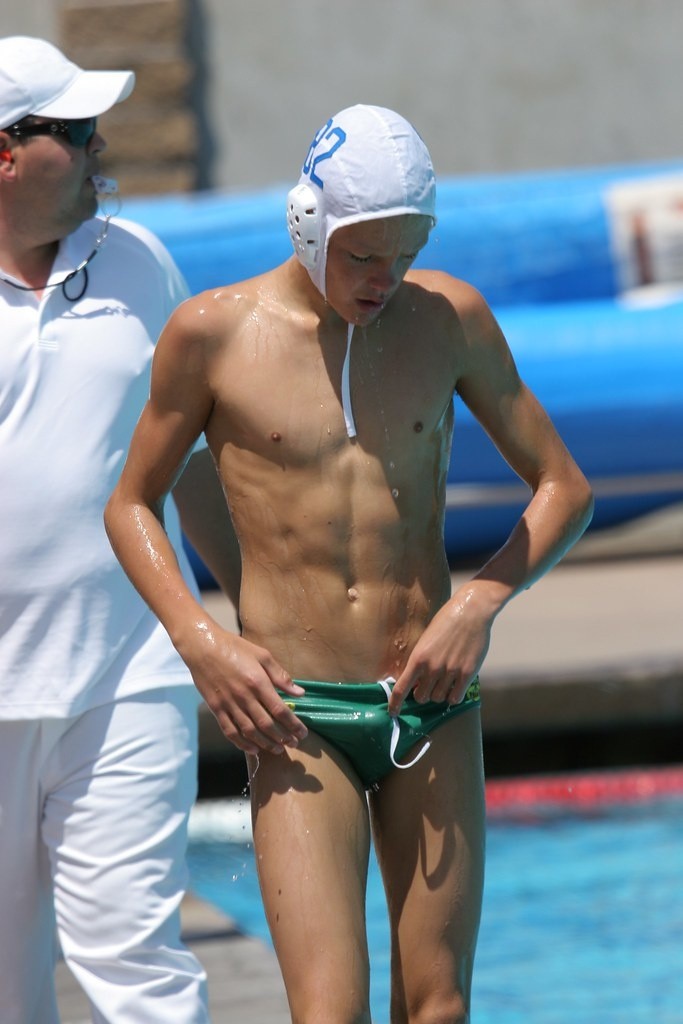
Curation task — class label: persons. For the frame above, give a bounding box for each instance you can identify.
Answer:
[0,33,199,1023]
[104,105,594,1024]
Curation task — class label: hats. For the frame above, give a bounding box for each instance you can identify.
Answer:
[286,104,435,302]
[0,36,136,132]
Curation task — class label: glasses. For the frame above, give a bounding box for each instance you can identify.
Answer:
[9,117,96,149]
